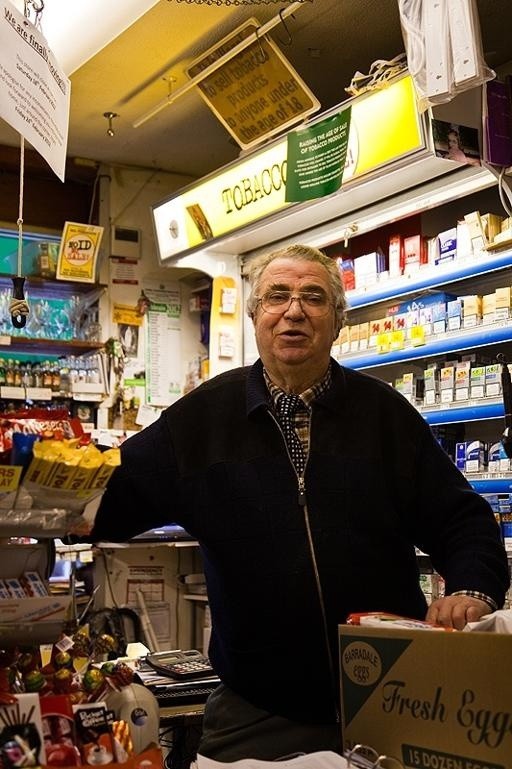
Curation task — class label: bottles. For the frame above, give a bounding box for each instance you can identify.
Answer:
[1,355,101,390]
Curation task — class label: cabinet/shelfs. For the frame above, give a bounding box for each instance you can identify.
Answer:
[2,174,512,697]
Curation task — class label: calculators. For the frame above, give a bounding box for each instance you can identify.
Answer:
[146,652,214,681]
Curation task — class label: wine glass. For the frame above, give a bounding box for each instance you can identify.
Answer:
[68,297,100,339]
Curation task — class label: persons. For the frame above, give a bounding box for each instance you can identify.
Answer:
[61,247,509,762]
[445,129,466,162]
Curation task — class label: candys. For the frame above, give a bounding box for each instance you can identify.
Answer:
[0,631,134,694]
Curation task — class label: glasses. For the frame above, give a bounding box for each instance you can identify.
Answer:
[254,291,337,321]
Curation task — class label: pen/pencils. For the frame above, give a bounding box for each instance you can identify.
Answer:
[0,704,35,727]
